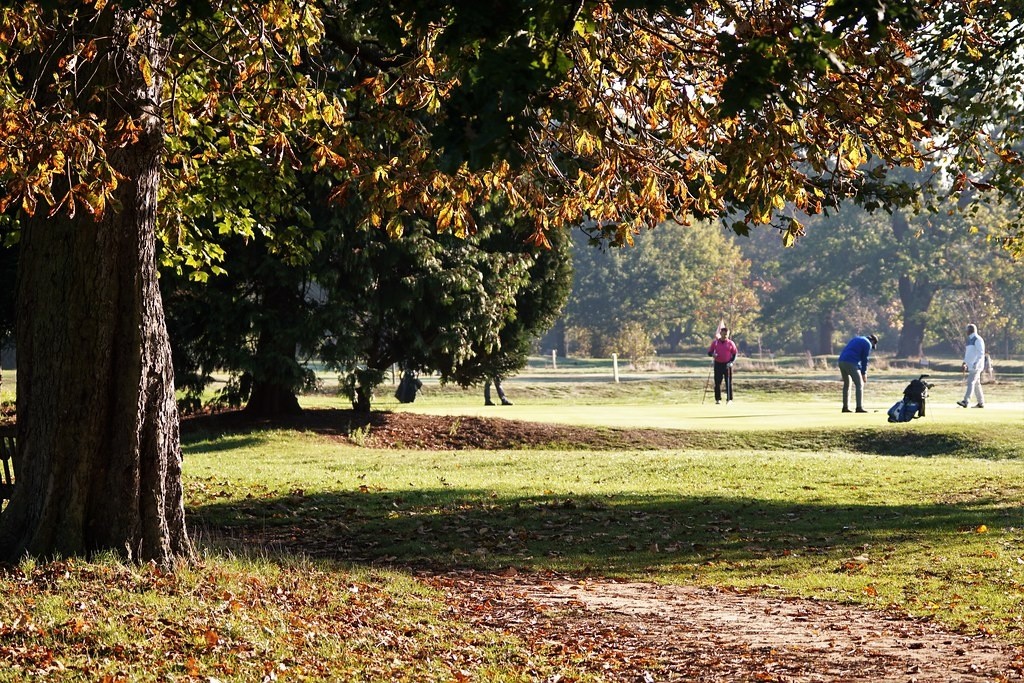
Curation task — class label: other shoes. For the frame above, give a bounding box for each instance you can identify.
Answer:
[486,401,495,405]
[726,400,732,405]
[502,401,513,405]
[971,403,984,408]
[842,408,852,412]
[716,400,720,404]
[957,401,968,408]
[856,408,866,412]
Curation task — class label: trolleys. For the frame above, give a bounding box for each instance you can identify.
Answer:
[887,375,935,423]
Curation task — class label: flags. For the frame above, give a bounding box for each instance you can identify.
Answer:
[716,320,725,339]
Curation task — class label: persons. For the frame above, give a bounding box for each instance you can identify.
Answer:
[956,323,985,409]
[837,334,878,413]
[984,351,993,379]
[707,327,738,405]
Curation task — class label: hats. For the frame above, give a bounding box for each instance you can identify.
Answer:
[869,334,878,349]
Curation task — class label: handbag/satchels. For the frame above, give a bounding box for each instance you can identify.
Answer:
[903,380,926,419]
